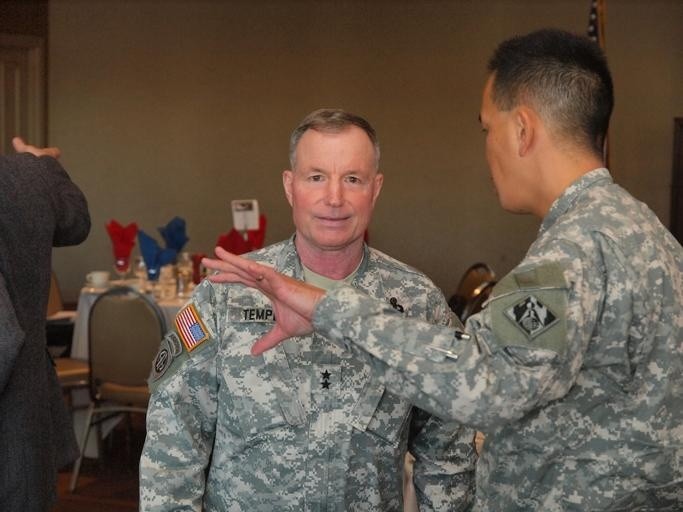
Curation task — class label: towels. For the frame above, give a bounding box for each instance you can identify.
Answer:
[104,213,268,285]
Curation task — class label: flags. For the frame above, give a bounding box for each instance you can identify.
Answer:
[584,1,609,170]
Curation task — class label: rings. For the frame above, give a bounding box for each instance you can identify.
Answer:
[255,275,264,282]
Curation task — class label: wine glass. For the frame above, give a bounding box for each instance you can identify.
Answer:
[116,252,192,305]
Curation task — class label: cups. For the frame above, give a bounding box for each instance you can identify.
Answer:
[87,272,111,287]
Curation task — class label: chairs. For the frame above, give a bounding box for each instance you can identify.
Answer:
[44,268,168,493]
[447,262,498,325]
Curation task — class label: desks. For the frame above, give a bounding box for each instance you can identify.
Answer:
[70,279,197,361]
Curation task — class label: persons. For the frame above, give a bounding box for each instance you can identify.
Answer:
[198,27,682,512]
[0,134,93,511]
[136,108,477,512]
